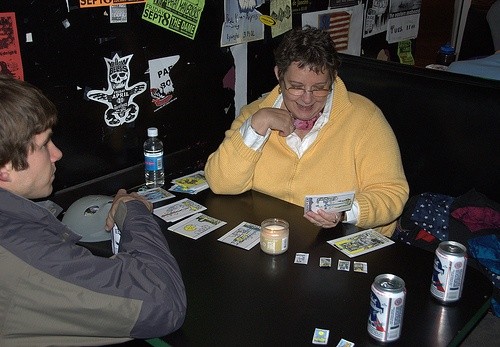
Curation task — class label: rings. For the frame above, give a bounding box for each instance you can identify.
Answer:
[333,215,339,223]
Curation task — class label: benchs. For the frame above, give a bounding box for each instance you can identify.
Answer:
[333,52,500,204]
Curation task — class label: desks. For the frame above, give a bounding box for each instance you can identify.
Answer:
[71,171,492,347]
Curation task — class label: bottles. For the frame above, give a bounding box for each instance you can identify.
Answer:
[440,41,454,66]
[143,127,165,188]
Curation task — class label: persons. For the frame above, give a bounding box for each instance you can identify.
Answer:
[0,76,188,347]
[204,25,409,238]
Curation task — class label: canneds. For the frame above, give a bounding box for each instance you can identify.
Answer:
[260,218,289,254]
[366,273,407,342]
[430,241,467,303]
[428,302,457,347]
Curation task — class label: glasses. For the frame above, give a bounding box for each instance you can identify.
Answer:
[282,75,332,97]
